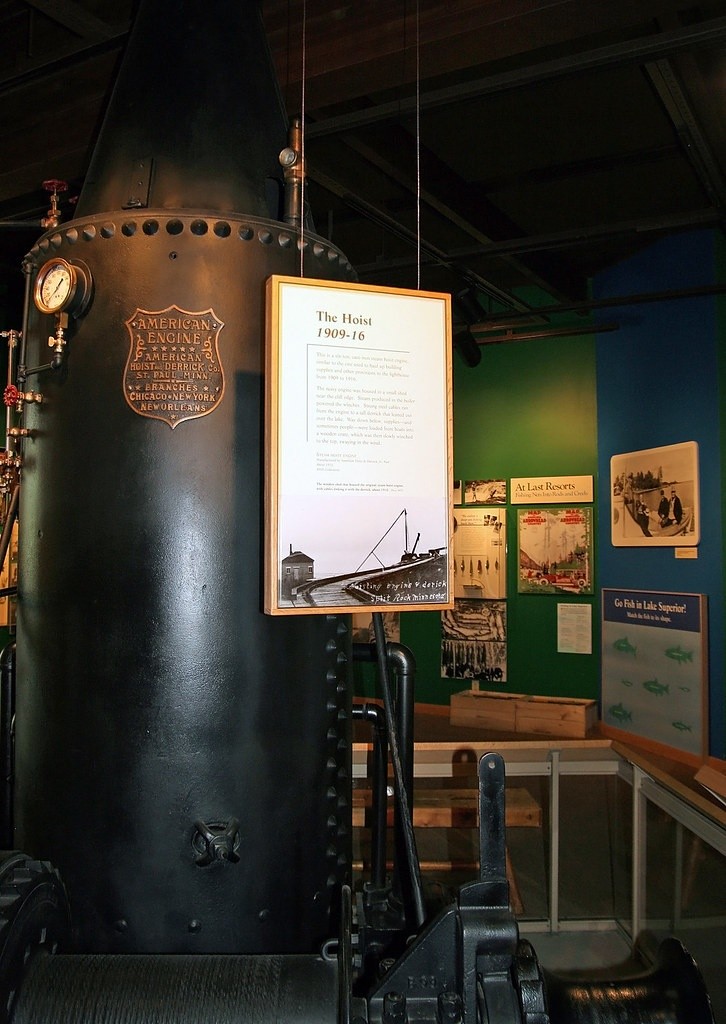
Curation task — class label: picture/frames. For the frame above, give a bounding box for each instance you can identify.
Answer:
[264,273,455,616]
[611,441,699,547]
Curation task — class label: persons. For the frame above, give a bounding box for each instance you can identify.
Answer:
[658,484,683,527]
[616,473,654,537]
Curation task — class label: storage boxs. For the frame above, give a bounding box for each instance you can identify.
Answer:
[449,689,597,739]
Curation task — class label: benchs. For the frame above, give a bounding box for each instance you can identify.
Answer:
[352,787,542,914]
